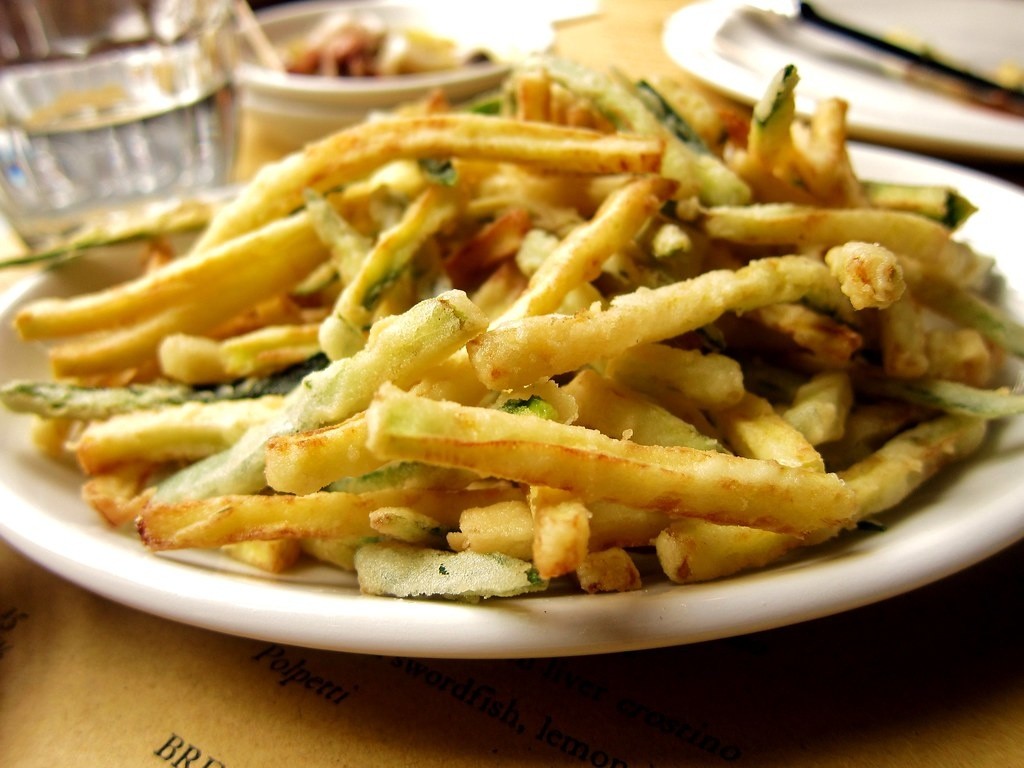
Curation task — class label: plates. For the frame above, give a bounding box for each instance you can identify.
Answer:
[663,0,1024,160]
[0,185,1024,660]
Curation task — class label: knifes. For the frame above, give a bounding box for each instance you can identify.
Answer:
[744,9,1024,118]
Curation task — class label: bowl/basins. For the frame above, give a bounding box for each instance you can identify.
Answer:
[220,0,556,152]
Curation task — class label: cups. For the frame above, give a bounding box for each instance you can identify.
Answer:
[0,0,242,248]
